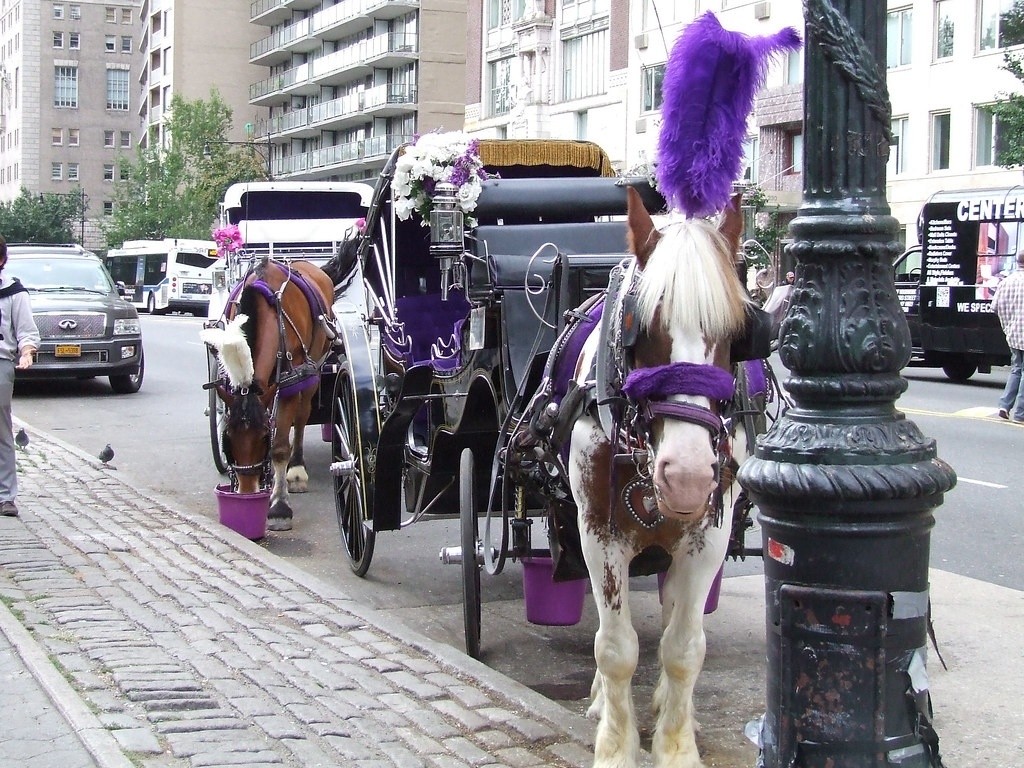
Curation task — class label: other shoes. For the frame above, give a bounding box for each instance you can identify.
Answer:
[0,501,19,517]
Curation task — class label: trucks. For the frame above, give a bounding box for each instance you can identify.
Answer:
[892,185,1024,381]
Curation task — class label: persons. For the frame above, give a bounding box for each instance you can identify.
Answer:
[0,234,42,515]
[780,271,795,285]
[990,251,1024,425]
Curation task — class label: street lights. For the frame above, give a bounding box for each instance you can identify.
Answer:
[203,131,277,182]
[38,187,88,247]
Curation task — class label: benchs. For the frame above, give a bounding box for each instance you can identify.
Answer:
[469,177,671,301]
[381,290,471,375]
[237,217,366,270]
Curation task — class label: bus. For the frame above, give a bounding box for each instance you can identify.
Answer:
[106,238,218,317]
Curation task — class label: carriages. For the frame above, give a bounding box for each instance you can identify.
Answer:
[203,181,374,532]
[329,137,771,768]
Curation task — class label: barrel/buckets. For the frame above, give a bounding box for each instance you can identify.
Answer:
[214,483,270,539]
[518,556,587,626]
[658,560,723,614]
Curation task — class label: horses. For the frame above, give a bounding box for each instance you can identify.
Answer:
[213,257,334,531]
[550,186,754,768]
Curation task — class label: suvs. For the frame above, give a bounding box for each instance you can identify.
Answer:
[2,242,144,393]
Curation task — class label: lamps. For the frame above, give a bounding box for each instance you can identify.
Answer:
[429,182,465,301]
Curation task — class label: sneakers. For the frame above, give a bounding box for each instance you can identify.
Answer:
[1013,420,1024,424]
[999,408,1010,420]
